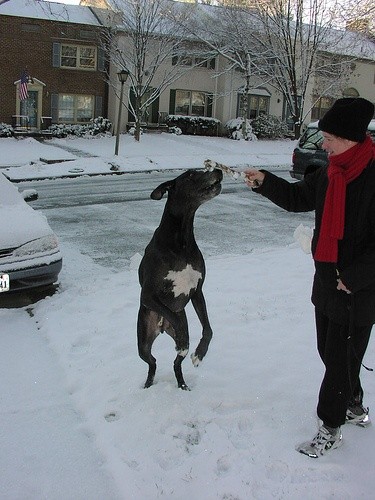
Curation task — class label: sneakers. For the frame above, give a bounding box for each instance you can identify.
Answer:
[298,426,345,458]
[345,406,371,428]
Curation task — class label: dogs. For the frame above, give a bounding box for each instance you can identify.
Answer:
[136,168,224,392]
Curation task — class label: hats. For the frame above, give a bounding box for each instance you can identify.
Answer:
[318,98,375,144]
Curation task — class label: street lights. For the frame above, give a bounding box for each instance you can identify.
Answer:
[115,70,130,155]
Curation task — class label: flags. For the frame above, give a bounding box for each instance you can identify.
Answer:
[19,70,29,100]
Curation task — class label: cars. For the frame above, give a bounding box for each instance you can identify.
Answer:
[288,119,375,180]
[0,171,63,296]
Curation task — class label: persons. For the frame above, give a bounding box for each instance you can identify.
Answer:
[242,97,373,459]
